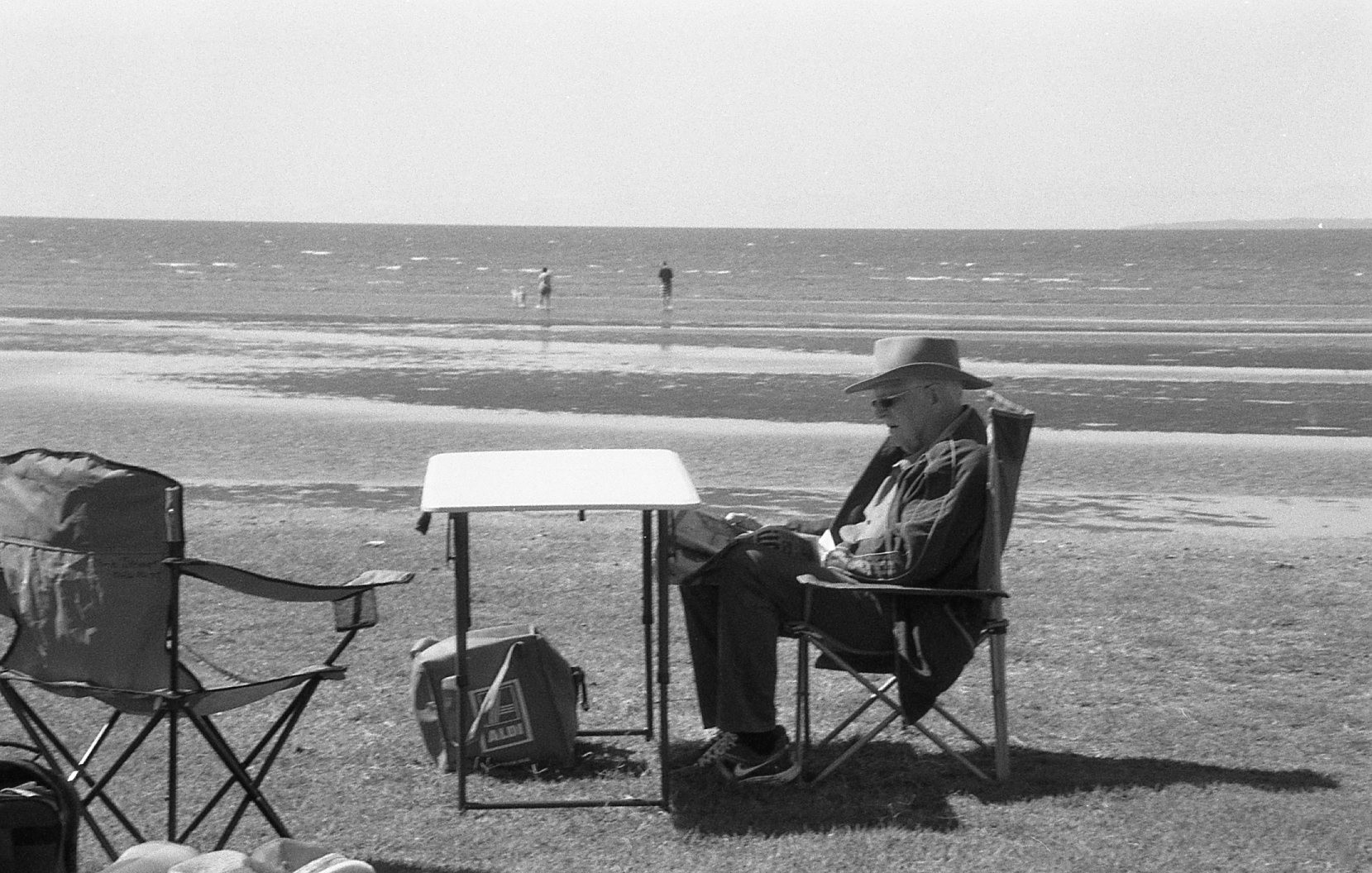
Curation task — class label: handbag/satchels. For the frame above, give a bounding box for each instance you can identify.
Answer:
[410,624,576,770]
[0,742,79,873]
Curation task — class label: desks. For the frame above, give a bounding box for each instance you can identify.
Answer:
[421,449,701,816]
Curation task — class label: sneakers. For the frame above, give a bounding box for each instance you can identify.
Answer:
[676,725,789,754]
[695,731,800,787]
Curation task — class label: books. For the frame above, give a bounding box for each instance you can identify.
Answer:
[665,508,740,555]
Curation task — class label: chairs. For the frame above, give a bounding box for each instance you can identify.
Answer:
[783,388,1034,793]
[0,447,415,867]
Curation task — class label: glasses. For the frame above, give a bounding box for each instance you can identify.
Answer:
[871,384,938,411]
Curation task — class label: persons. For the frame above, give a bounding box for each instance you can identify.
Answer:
[537,267,552,305]
[659,262,673,297]
[682,337,992,782]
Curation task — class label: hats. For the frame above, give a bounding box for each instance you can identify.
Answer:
[844,336,992,393]
